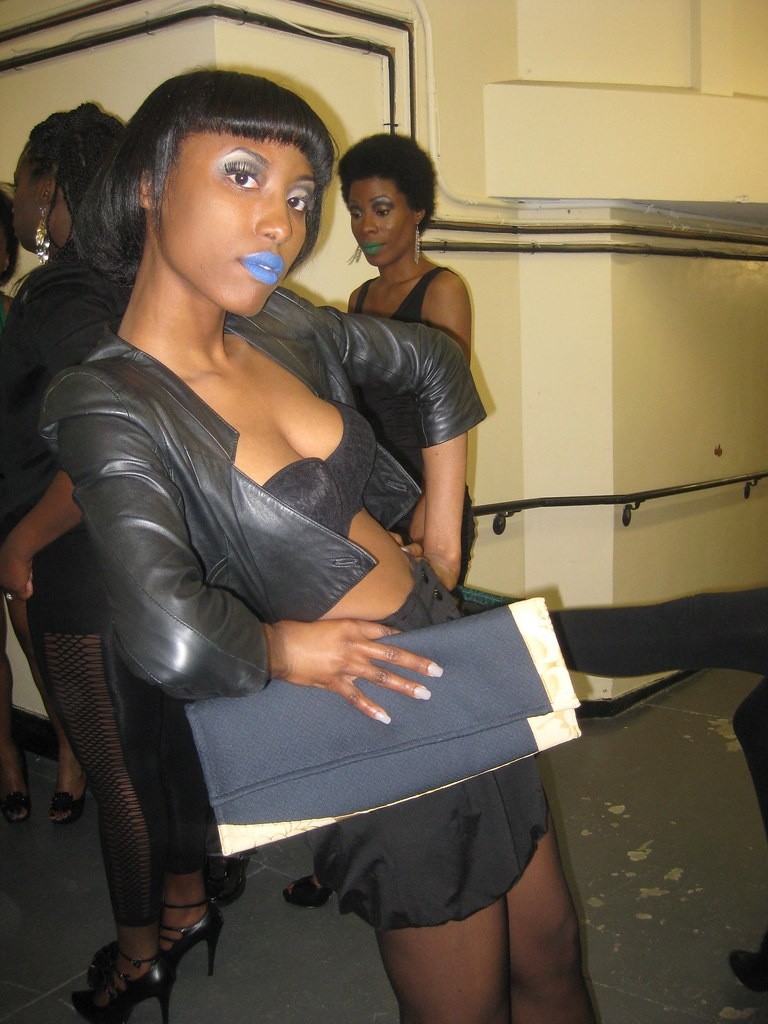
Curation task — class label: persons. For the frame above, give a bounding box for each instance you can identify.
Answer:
[0,98,474,1024]
[728,674,768,993]
[37,69,600,1024]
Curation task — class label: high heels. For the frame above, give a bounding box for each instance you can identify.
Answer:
[154,898,223,978]
[70,941,177,1024]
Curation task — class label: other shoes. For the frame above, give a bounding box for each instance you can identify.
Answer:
[729,937,768,992]
[49,776,87,824]
[202,854,246,905]
[282,875,334,907]
[0,790,31,823]
